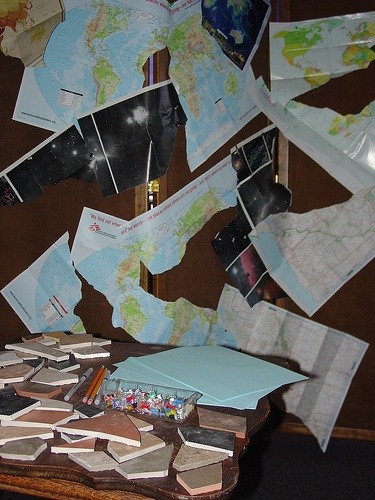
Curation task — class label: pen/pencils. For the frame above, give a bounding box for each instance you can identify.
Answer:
[94,370,111,405]
[82,364,105,403]
[63,367,94,401]
[87,367,108,405]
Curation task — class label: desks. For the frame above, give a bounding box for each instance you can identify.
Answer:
[0,330,301,500]
